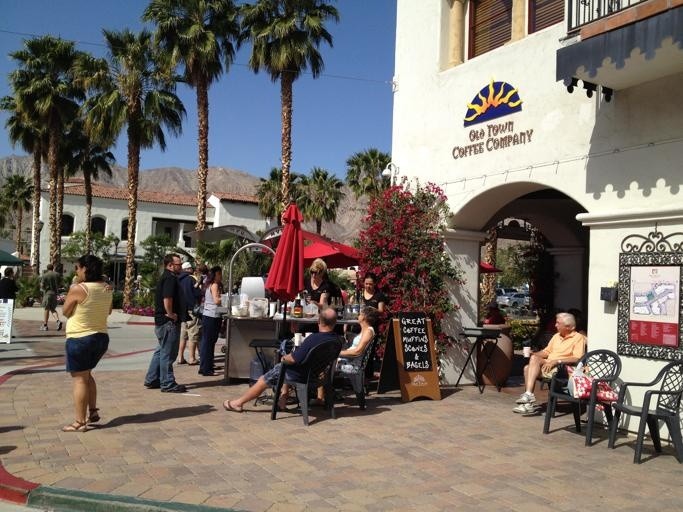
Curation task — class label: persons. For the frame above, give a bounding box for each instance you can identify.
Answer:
[0,268,18,338]
[177,262,224,375]
[63,255,113,432]
[512,312,585,413]
[482,302,505,324]
[40,265,62,331]
[144,253,186,392]
[222,258,385,413]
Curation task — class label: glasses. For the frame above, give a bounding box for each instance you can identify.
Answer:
[309,270,322,276]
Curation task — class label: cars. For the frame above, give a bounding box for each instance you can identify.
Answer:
[493,284,530,309]
[344,302,358,312]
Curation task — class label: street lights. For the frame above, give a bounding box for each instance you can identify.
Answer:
[35,219,44,273]
[113,235,120,291]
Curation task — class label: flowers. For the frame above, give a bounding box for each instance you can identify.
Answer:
[479,300,514,324]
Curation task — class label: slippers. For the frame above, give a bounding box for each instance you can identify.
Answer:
[223,399,243,413]
[277,405,289,412]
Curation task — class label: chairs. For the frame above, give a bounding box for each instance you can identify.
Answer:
[247,328,384,428]
[522,345,683,466]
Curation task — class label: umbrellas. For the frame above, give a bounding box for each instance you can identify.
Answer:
[264,203,304,340]
[0,250,23,267]
[252,228,363,269]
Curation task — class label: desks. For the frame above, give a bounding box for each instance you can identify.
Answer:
[449,332,506,395]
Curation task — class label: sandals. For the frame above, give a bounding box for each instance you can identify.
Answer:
[85,408,101,423]
[61,420,88,433]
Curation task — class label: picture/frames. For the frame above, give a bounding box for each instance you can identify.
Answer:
[616,250,683,362]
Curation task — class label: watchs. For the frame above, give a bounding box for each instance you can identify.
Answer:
[557,360,562,364]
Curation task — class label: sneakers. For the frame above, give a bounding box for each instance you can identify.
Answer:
[55,321,62,331]
[514,393,537,405]
[143,382,160,388]
[39,325,48,331]
[511,402,536,414]
[160,384,187,393]
[176,353,223,377]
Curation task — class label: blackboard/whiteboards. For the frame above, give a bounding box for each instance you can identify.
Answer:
[400,311,433,372]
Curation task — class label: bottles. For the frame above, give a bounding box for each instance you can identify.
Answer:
[293,287,368,320]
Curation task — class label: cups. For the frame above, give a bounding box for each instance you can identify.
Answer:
[295,333,302,347]
[522,346,531,357]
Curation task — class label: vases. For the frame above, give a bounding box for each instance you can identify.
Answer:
[480,321,516,387]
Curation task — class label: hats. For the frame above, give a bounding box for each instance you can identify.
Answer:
[181,261,193,270]
